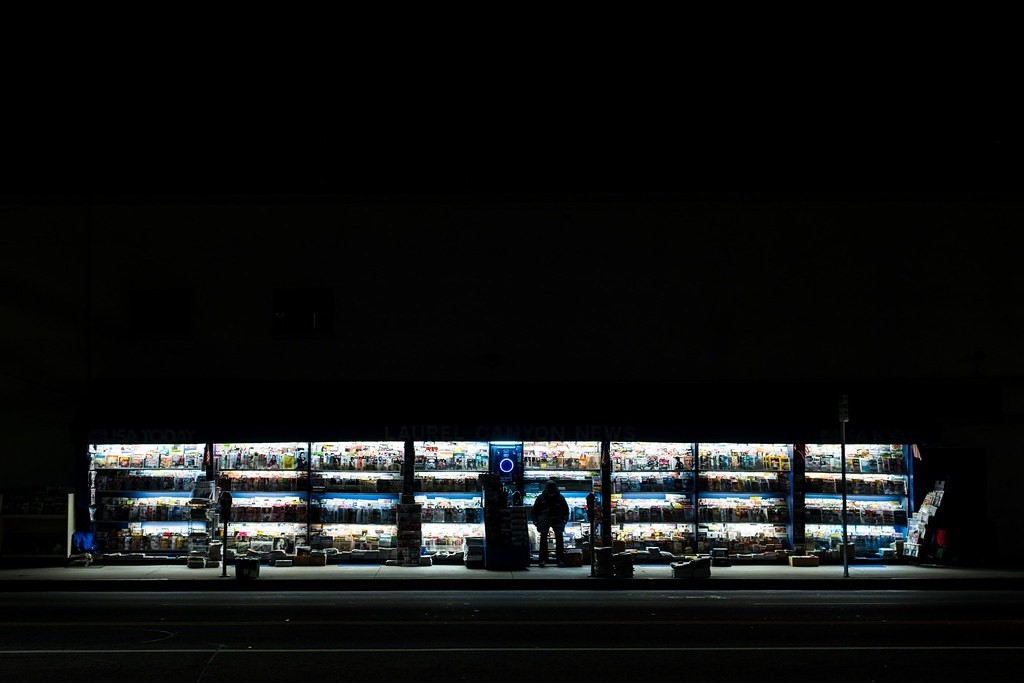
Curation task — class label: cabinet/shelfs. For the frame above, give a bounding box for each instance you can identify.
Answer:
[88,442,917,565]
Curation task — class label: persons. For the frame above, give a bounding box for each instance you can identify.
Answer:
[532,479,569,568]
